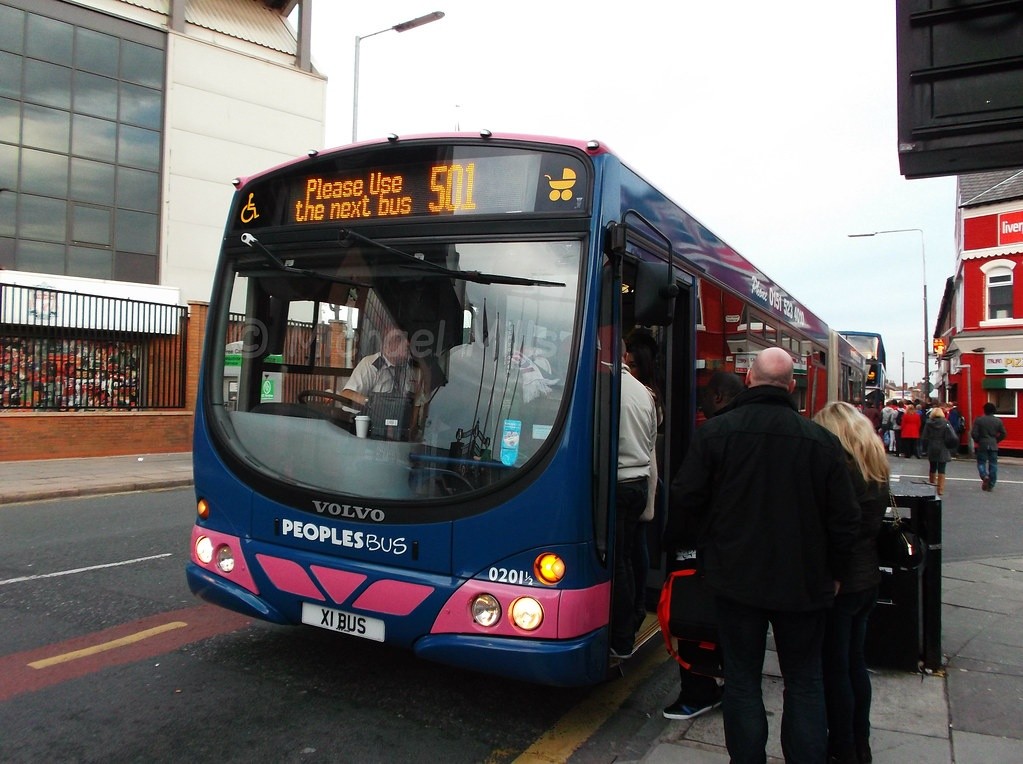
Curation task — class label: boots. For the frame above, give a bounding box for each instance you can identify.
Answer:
[929,471,937,483]
[937,473,945,494]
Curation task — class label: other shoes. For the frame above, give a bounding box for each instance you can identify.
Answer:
[856,735,874,764]
[826,738,859,764]
[982,476,993,492]
[608,648,631,660]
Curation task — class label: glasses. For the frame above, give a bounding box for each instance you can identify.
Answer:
[626,361,639,369]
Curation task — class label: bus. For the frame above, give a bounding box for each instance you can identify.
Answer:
[838,331,886,406]
[185,126,870,685]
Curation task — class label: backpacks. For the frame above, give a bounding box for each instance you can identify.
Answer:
[895,409,904,425]
[957,414,966,434]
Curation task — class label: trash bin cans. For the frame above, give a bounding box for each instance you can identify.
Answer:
[871,493,943,673]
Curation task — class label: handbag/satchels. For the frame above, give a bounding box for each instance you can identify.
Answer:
[658,567,728,677]
[944,421,960,449]
[886,415,893,431]
[883,432,890,445]
[877,488,928,574]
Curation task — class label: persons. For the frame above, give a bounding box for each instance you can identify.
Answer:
[921,407,951,494]
[611,326,663,659]
[811,401,890,764]
[671,347,861,764]
[971,403,1005,492]
[342,329,425,439]
[663,372,745,719]
[882,398,960,460]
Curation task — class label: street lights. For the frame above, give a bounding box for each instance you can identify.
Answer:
[847,228,930,403]
[345,10,446,379]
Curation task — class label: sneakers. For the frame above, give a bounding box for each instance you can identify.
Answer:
[663,690,722,720]
